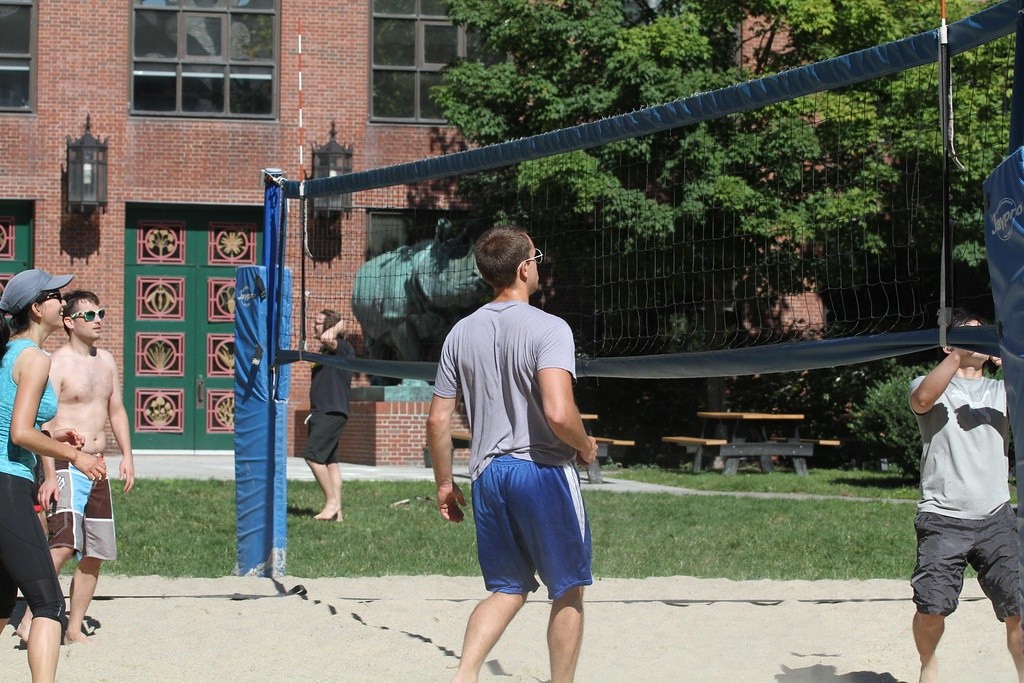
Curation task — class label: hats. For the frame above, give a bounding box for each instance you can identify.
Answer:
[0,269,74,314]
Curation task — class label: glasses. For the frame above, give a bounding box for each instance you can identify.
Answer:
[71,309,105,322]
[37,292,62,304]
[516,249,542,271]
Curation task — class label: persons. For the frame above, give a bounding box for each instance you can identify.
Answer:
[426,227,599,683]
[907,310,1024,683]
[303,309,356,522]
[0,270,137,683]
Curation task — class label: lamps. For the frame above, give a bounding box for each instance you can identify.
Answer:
[311,117,353,219]
[65,113,111,213]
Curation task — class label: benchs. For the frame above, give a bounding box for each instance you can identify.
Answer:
[595,437,635,445]
[773,437,841,445]
[663,436,727,445]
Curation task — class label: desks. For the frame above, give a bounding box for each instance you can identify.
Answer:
[580,413,608,484]
[686,412,813,477]
